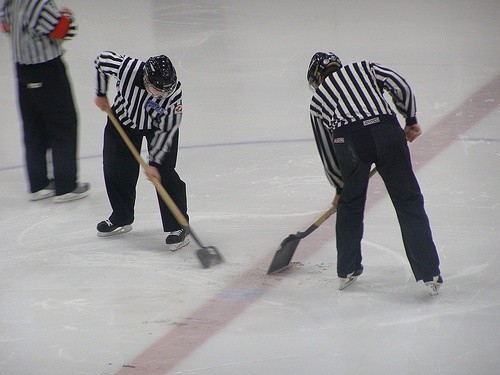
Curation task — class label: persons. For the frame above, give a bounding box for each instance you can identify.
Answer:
[94,50,190,250]
[307,50,444,295]
[0,0,92,201]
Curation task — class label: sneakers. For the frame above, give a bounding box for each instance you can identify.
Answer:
[54,181,90,202]
[166,229,190,251]
[336,263,363,290]
[96,216,133,238]
[30,178,54,200]
[423,275,443,296]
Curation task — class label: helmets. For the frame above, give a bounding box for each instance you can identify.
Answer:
[307,52,342,82]
[144,55,177,92]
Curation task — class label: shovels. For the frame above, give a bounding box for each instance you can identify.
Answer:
[102,104,225,269]
[266,129,416,275]
